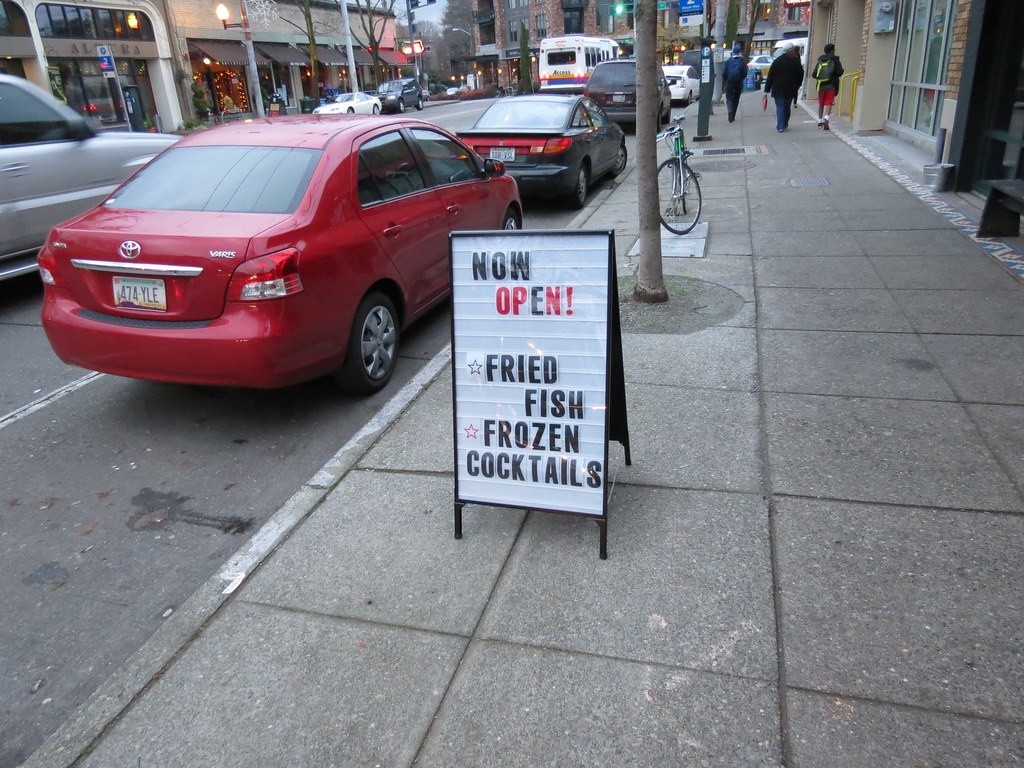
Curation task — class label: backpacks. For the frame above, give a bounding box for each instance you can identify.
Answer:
[728,57,744,81]
[816,57,834,82]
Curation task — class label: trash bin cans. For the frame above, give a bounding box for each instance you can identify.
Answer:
[299,96,321,114]
[746,68,761,91]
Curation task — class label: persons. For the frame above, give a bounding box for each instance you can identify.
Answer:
[764,42,804,132]
[794,46,801,108]
[722,46,747,122]
[321,81,331,99]
[812,44,844,131]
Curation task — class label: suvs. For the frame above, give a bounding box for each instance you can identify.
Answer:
[372,78,424,114]
[584,56,677,133]
[0,73,187,280]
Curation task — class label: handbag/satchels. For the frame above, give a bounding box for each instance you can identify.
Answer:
[762,94,767,111]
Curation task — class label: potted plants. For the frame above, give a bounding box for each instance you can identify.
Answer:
[145,112,157,133]
[190,82,210,123]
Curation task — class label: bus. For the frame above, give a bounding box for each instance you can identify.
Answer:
[538,36,620,97]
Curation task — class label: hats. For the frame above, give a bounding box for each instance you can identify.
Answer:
[733,45,741,54]
[782,41,794,52]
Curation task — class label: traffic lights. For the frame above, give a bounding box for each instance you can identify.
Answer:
[400,45,413,56]
[608,5,625,15]
[414,39,424,54]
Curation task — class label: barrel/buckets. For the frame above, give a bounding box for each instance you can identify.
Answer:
[923,162,955,193]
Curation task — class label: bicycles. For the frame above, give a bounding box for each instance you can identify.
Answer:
[655,116,703,236]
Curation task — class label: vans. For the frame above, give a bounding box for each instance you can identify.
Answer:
[769,36,808,68]
[680,49,734,80]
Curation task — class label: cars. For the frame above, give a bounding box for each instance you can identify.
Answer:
[421,90,430,102]
[459,86,471,93]
[662,65,700,106]
[453,94,628,210]
[746,55,777,82]
[446,88,459,97]
[38,114,525,397]
[312,92,382,115]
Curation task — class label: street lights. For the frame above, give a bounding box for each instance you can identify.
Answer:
[217,0,265,119]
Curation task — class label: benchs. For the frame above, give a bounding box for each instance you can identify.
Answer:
[975,178,1024,238]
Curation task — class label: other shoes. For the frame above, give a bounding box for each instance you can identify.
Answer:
[728,111,735,122]
[779,129,784,132]
[823,119,829,130]
[818,122,823,128]
[793,105,797,108]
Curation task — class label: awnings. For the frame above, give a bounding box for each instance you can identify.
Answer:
[297,44,349,65]
[187,38,268,65]
[253,41,311,65]
[335,44,384,66]
[369,47,413,66]
